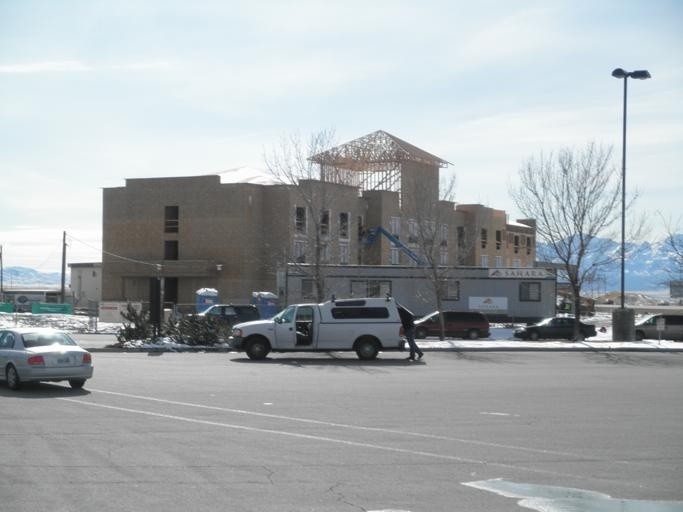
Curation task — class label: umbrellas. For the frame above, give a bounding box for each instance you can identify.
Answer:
[197,286,218,297]
[253,291,280,302]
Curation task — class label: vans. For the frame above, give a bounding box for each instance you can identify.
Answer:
[413,310,490,339]
[229,298,407,361]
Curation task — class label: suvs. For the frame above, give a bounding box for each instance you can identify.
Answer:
[183,303,259,342]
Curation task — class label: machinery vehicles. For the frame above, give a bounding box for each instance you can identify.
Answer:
[362,226,427,266]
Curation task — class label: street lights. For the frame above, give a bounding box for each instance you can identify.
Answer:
[611,68,651,308]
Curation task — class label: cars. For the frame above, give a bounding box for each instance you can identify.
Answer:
[513,318,596,340]
[0,327,94,391]
[633,314,682,342]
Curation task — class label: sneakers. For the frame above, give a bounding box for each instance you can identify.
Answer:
[407,353,423,359]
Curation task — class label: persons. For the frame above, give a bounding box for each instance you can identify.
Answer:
[397,305,424,362]
